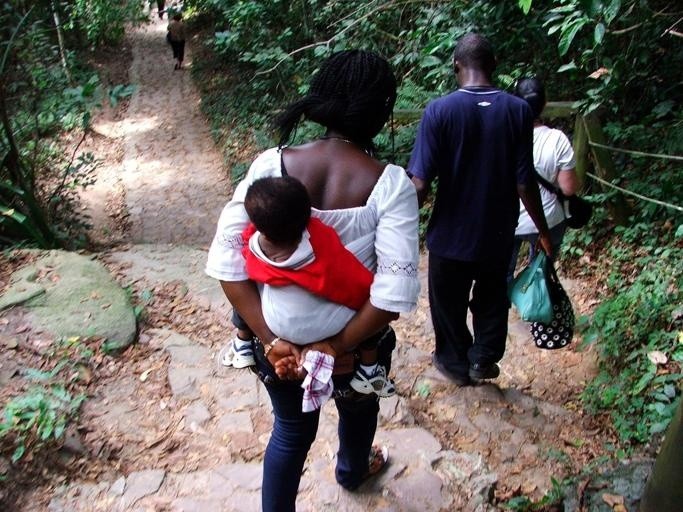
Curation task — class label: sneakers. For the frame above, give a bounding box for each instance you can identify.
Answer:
[469,363,499,378]
[350,367,395,397]
[222,341,255,368]
[174,64,181,70]
[432,350,470,385]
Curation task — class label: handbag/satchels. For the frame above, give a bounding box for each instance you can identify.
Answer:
[530,257,574,348]
[508,250,553,325]
[559,189,592,228]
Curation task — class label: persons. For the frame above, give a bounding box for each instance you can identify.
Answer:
[140,0,188,70]
[217,175,398,398]
[203,49,420,511]
[405,32,553,386]
[504,74,580,311]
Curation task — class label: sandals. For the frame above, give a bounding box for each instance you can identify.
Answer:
[368,443,388,476]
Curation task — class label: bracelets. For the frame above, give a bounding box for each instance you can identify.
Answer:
[262,334,282,361]
